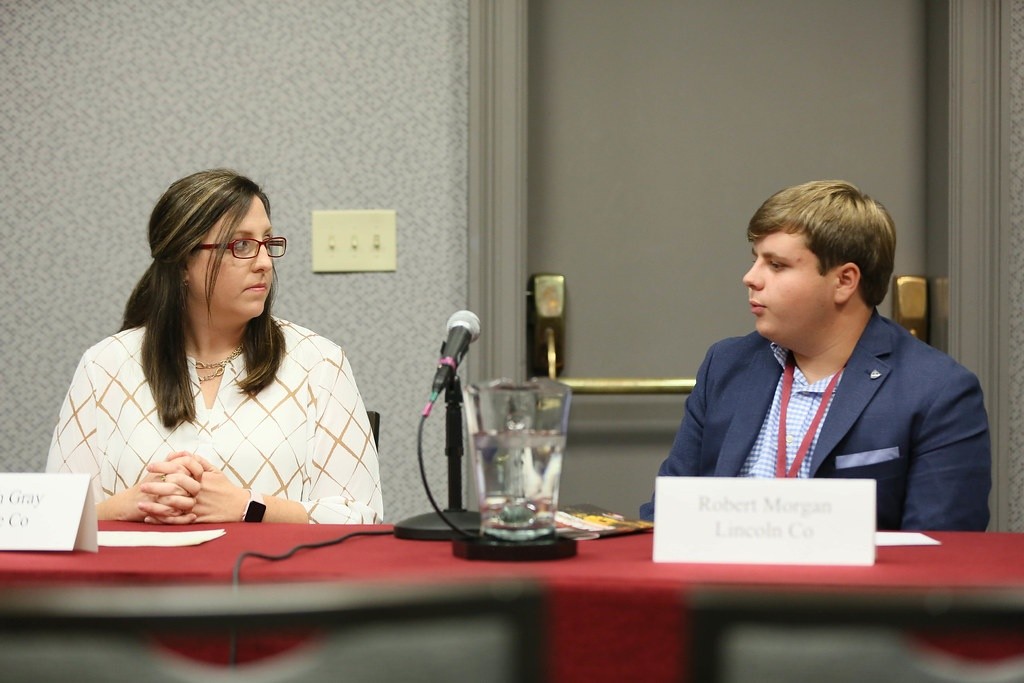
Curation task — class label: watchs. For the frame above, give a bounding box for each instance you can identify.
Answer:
[241,489,267,522]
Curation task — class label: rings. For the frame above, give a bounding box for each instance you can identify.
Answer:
[160,473,167,482]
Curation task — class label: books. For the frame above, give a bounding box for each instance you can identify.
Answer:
[554,503,654,541]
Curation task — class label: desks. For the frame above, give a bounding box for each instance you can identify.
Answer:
[0,519,1024,683]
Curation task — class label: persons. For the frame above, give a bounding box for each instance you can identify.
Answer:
[640,180,991,532]
[46,169,384,525]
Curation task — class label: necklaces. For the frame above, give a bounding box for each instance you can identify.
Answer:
[196,340,243,383]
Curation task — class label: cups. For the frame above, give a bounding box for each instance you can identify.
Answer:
[463,379,572,540]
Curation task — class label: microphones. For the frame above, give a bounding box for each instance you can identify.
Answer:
[423,310,481,419]
